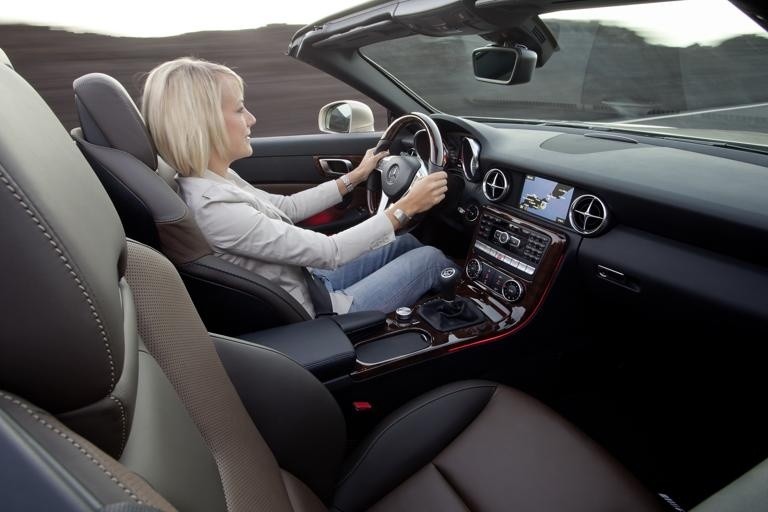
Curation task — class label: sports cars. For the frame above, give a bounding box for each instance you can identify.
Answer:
[1,1,768,505]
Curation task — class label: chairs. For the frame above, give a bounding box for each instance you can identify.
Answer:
[72,71,310,327]
[0,45,651,512]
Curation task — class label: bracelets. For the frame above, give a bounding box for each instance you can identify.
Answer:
[338,174,355,196]
[387,201,412,228]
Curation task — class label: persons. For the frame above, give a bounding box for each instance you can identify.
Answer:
[141,58,463,319]
